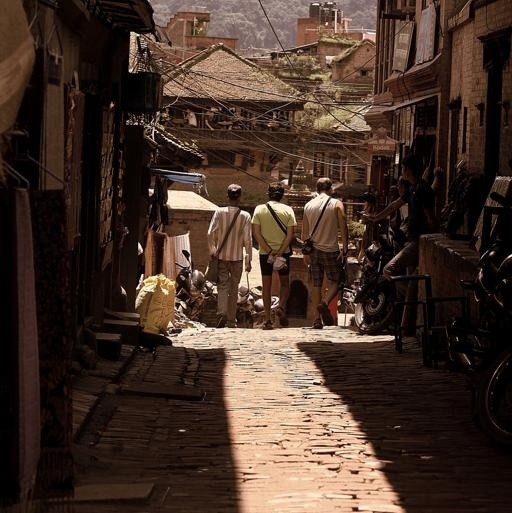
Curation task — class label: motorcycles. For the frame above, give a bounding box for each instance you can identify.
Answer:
[235,285,280,328]
[175,250,216,322]
[444,192,512,449]
[353,210,396,333]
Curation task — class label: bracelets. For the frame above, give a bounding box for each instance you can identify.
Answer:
[342,249,348,256]
[268,248,272,255]
[245,253,250,257]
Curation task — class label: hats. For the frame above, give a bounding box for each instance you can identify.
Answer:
[316,176,331,190]
[227,182,241,199]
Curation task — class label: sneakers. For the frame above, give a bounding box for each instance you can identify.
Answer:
[216,313,225,327]
[311,302,334,328]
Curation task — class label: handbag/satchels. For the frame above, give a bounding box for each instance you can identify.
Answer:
[203,255,219,282]
[300,237,312,255]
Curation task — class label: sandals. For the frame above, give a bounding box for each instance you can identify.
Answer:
[262,307,288,329]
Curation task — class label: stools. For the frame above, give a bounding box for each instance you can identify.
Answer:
[389,274,433,354]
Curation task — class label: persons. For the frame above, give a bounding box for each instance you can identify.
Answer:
[398,165,445,238]
[204,106,223,130]
[250,182,298,330]
[301,176,349,329]
[207,183,253,328]
[182,107,198,128]
[161,107,176,127]
[361,152,437,307]
